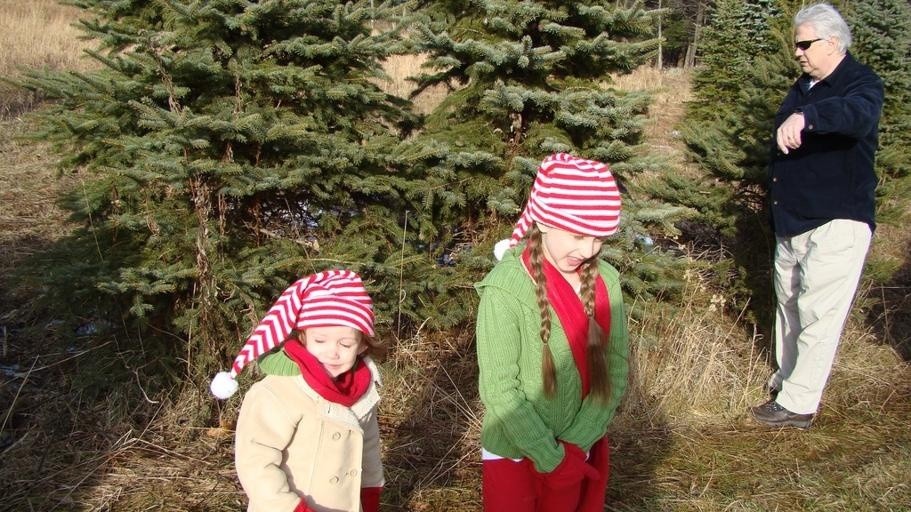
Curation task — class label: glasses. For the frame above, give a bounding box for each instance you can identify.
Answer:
[796,38,824,52]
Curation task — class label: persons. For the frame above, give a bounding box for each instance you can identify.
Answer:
[746,4,884,427]
[474,153,630,512]
[210,270,386,512]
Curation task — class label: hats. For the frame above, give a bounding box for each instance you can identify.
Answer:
[494,154,621,261]
[208,270,375,400]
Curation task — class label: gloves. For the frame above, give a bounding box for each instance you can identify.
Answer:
[528,442,587,480]
[360,487,384,512]
[294,498,315,512]
[546,452,601,488]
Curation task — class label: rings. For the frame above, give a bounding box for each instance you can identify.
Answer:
[788,136,794,139]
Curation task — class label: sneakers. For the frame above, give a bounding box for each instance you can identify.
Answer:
[749,402,811,428]
